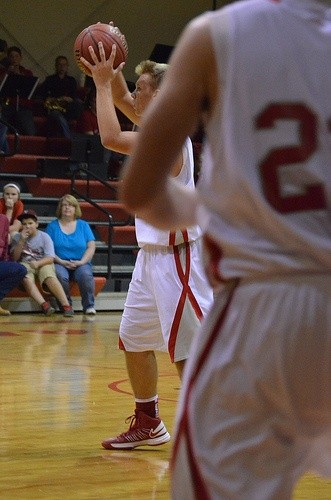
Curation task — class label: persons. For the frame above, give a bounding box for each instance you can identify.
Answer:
[118,0,331,500]
[0,38,122,181]
[0,182,96,316]
[78,20,213,451]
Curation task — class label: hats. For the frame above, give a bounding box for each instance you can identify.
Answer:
[17,209,38,221]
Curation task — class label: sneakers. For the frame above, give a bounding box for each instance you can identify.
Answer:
[102,409,170,450]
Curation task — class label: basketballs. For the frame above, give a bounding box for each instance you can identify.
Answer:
[72,23,130,78]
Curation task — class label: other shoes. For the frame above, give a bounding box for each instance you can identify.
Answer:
[85,308,96,315]
[62,305,74,316]
[0,307,11,316]
[40,302,55,316]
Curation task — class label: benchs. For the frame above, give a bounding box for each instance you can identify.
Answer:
[0,86,210,298]
